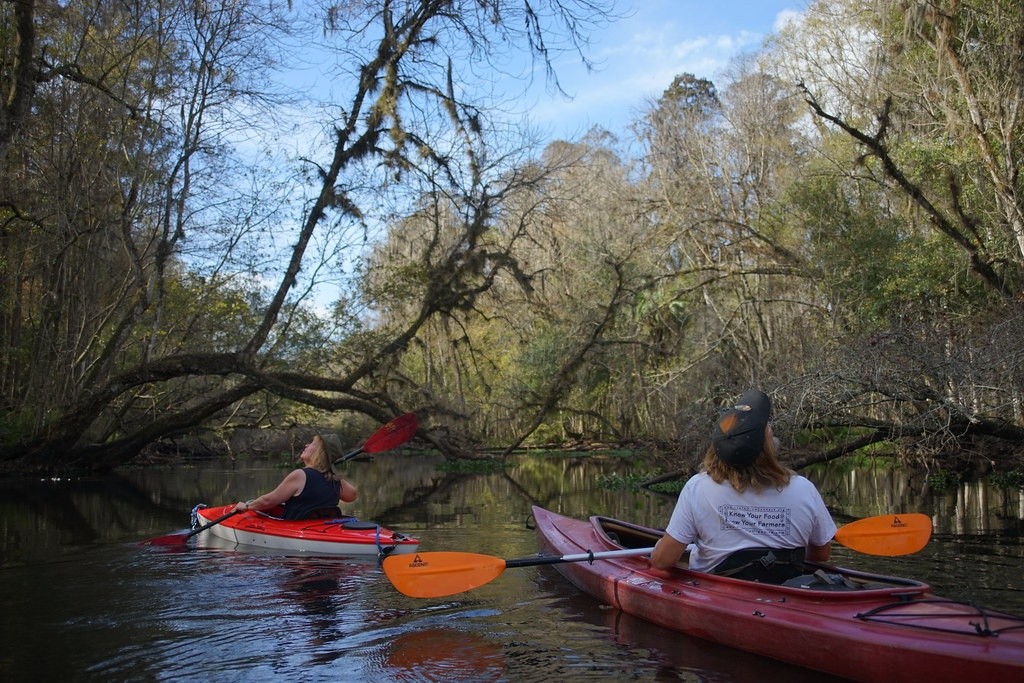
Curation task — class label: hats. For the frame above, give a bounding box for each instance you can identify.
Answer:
[713,390,772,467]
[312,425,345,475]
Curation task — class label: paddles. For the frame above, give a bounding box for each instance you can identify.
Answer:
[138,409,421,547]
[381,511,933,600]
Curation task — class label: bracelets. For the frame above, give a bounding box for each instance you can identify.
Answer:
[246,504,248,509]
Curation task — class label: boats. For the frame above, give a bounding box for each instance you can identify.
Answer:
[532,504,1024,683]
[196,501,421,559]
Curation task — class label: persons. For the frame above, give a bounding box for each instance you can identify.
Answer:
[234,428,356,520]
[651,390,837,586]
